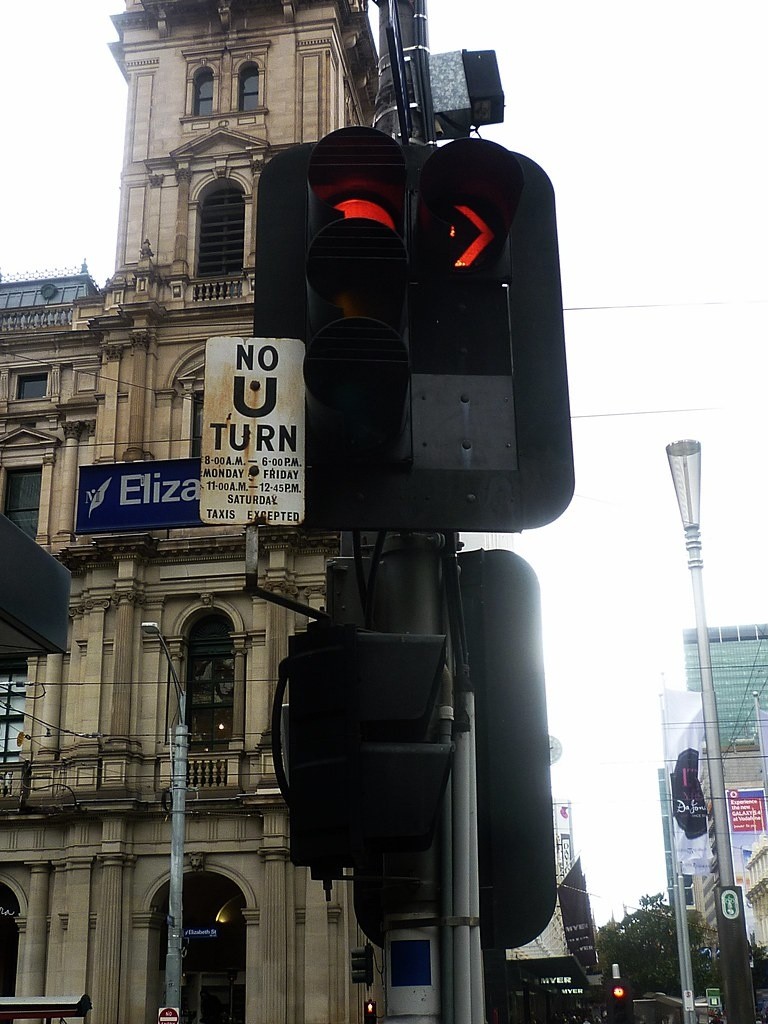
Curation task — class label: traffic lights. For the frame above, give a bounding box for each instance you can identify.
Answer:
[309,629,456,870]
[613,986,626,1015]
[350,943,374,989]
[363,999,377,1024]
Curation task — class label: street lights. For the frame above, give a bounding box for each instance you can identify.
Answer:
[141,621,189,1008]
[653,437,757,1024]
[252,125,578,536]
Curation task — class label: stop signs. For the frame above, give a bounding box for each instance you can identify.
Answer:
[157,1006,179,1024]
